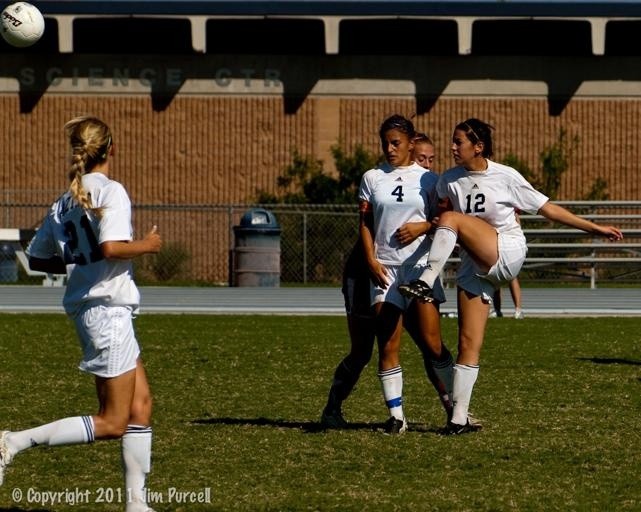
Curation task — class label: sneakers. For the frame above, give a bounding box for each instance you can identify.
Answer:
[0,430,14,486]
[322,408,348,424]
[383,416,408,436]
[489,311,503,317]
[436,413,483,435]
[513,311,524,319]
[398,279,434,303]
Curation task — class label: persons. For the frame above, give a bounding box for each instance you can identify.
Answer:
[0,116,162,511]
[317,111,624,434]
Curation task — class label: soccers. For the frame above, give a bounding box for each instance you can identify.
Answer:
[1,2,45,47]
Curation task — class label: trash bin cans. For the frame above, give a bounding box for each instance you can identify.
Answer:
[233,208,283,287]
